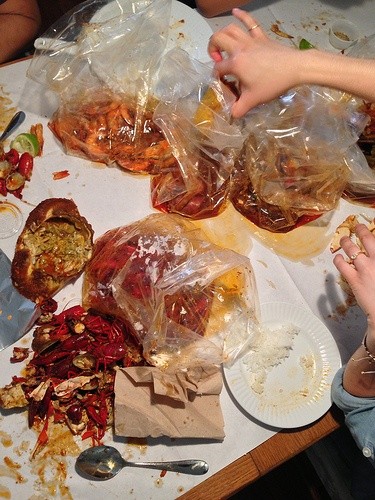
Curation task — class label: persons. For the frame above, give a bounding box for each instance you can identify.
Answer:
[0,0,42,65]
[207,6,375,460]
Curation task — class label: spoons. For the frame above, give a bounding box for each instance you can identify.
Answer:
[77,446,209,480]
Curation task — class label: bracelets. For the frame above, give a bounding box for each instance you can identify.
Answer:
[360,330,375,375]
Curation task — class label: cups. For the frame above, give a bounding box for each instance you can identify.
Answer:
[329,20,360,49]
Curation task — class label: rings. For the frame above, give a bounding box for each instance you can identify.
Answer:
[350,251,367,265]
[248,24,260,31]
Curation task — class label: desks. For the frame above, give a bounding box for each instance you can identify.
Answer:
[0,0,375,500]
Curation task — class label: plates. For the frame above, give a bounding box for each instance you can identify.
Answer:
[223,302,341,428]
[88,0,214,89]
